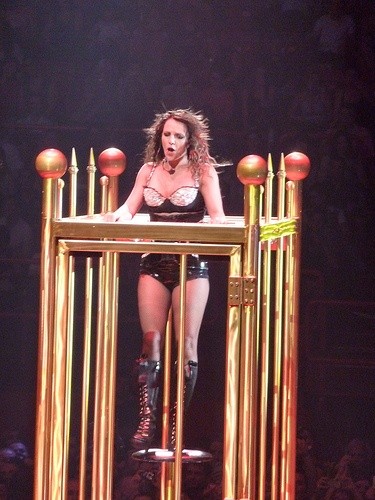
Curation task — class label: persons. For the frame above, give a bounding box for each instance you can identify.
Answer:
[101,99,240,451]
[0,431,375,500]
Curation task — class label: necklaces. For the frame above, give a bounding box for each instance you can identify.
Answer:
[161,158,188,174]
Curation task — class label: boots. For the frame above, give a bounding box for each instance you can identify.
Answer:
[169,361,199,453]
[129,357,161,447]
